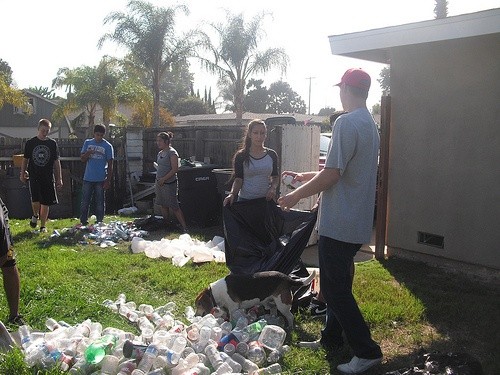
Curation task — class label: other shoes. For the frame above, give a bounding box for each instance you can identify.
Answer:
[300,338,322,349]
[30,215,38,228]
[307,297,327,317]
[336,356,384,375]
[8,315,24,325]
[40,227,48,232]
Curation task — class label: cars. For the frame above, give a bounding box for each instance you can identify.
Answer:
[318,132,332,171]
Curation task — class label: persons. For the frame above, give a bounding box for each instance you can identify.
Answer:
[20,119,63,233]
[75,124,114,228]
[0,196,25,328]
[223,119,279,206]
[305,110,349,319]
[153,131,190,234]
[277,67,383,374]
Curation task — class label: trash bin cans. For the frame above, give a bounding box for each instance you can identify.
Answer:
[213,168,236,223]
[176,163,224,228]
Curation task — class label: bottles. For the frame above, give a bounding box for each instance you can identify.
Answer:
[54,229,61,238]
[17,294,292,375]
[115,228,129,240]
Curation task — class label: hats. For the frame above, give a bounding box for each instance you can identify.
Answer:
[334,68,371,91]
